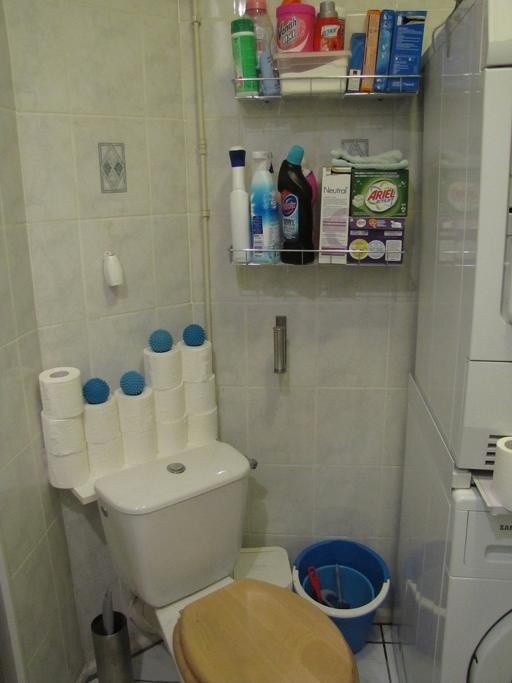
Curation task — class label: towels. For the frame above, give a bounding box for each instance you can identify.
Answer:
[330,149,409,171]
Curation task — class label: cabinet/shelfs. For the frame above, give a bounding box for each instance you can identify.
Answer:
[229,58,423,269]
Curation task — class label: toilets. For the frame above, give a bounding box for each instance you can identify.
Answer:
[92,439,361,683]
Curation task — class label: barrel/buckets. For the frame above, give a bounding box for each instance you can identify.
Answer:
[290,533,391,656]
